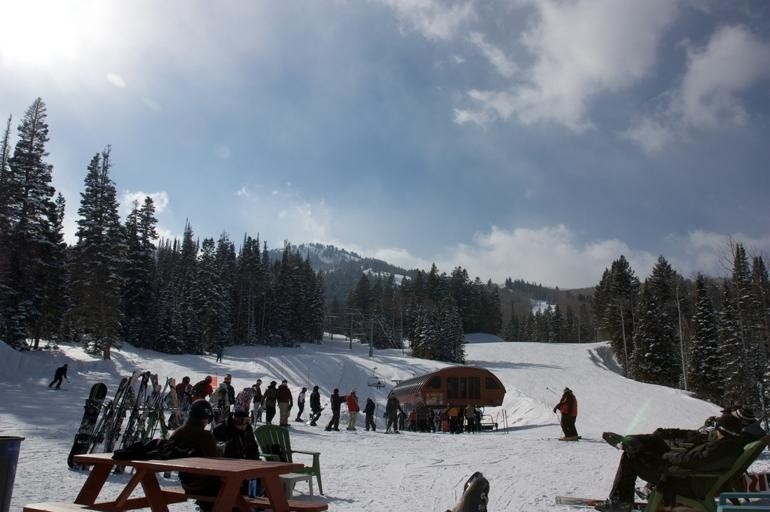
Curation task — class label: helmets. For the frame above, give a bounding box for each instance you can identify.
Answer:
[190,399,213,424]
[224,374,231,382]
[205,376,211,383]
[232,411,250,425]
[182,376,190,383]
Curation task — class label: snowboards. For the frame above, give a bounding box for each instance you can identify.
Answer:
[208,383,231,430]
[232,388,258,415]
[69,383,107,470]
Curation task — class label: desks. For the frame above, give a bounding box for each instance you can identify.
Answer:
[73,451,304,511]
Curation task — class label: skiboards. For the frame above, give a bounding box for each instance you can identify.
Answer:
[559,437,582,441]
[554,495,649,511]
[47,388,69,391]
[82,371,194,472]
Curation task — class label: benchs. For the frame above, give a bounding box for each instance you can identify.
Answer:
[22,502,100,512]
[160,485,328,511]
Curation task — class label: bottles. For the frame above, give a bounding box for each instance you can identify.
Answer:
[249,477,257,500]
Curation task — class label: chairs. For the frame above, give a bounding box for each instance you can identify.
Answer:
[254,424,324,493]
[715,492,770,512]
[645,435,768,512]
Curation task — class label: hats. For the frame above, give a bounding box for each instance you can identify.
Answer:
[714,404,757,439]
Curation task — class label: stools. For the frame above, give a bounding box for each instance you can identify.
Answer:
[279,473,314,499]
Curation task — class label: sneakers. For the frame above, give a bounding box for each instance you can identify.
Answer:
[595,497,632,512]
[450,477,489,512]
[602,432,624,449]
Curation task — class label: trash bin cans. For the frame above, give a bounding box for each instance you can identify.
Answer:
[0,436,25,512]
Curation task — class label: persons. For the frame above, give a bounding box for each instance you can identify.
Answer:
[168,374,294,431]
[48,363,71,390]
[169,398,240,511]
[594,405,756,511]
[213,409,268,511]
[296,386,322,426]
[326,388,376,431]
[552,386,578,438]
[215,350,222,363]
[386,393,402,433]
[398,402,483,434]
